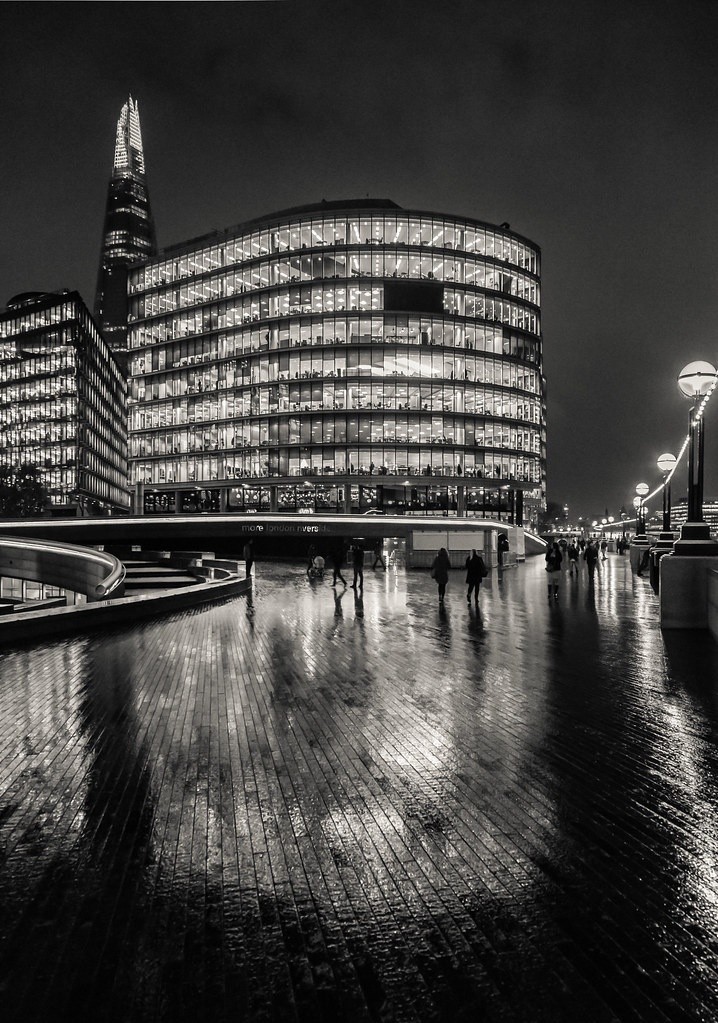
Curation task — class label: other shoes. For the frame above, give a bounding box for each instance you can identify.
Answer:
[467,594,471,602]
[438,599,443,606]
[475,598,479,604]
[547,593,551,598]
[553,593,558,599]
[576,570,578,573]
[602,557,609,560]
[349,584,356,589]
[344,582,348,587]
[570,571,573,575]
[358,585,362,589]
[330,584,336,587]
[589,576,595,582]
[383,567,387,570]
[371,567,375,570]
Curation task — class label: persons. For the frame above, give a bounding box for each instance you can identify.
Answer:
[244,536,258,578]
[465,548,489,605]
[303,539,388,588]
[544,536,630,600]
[430,547,451,603]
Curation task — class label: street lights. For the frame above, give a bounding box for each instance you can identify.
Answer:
[633,482,650,545]
[671,360,718,555]
[621,513,629,541]
[651,453,676,550]
[592,519,607,541]
[608,517,614,542]
